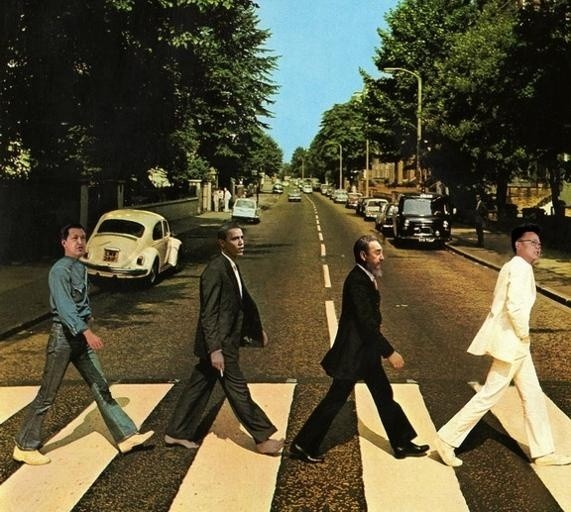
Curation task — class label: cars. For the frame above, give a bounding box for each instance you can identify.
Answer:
[77,207,186,290]
[272,176,455,248]
[230,198,262,222]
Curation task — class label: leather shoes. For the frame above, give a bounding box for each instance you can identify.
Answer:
[257,438,284,454]
[290,441,325,463]
[394,441,429,459]
[535,455,571,466]
[165,434,199,449]
[118,430,155,453]
[13,446,51,466]
[434,436,463,466]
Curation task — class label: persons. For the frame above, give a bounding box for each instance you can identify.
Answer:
[291,235,430,462]
[224,188,232,210]
[430,222,571,465]
[473,194,489,247]
[9,224,154,465]
[164,222,285,454]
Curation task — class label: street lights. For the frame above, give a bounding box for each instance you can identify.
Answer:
[329,141,343,189]
[384,67,424,191]
[297,155,304,181]
[351,127,370,197]
